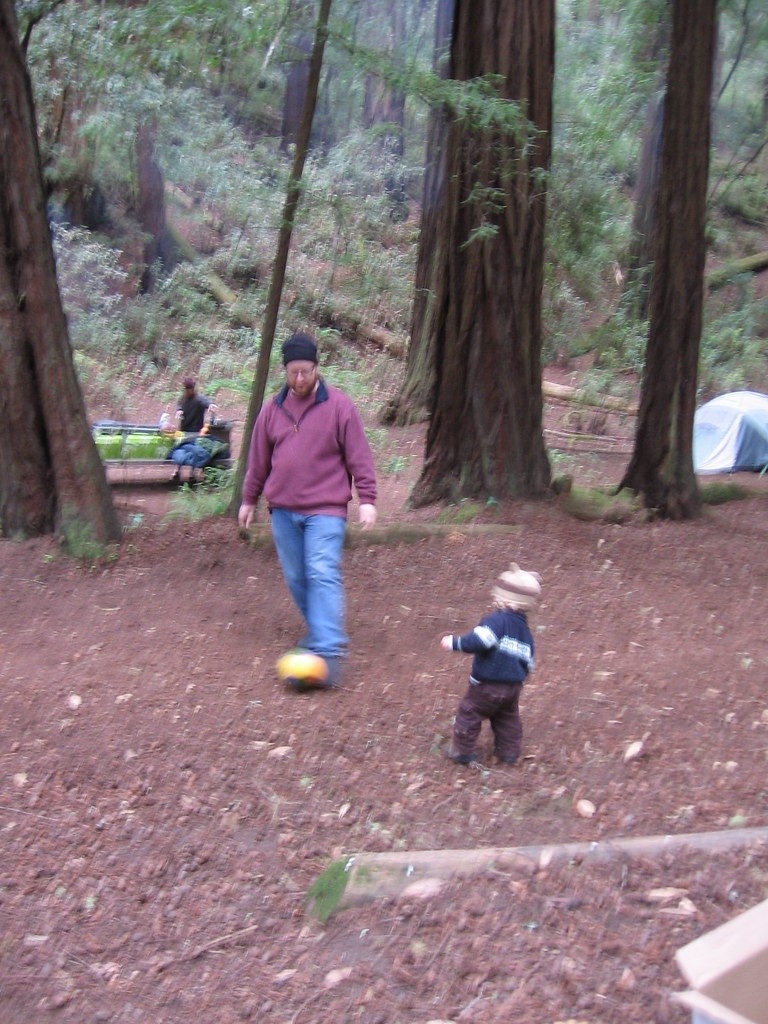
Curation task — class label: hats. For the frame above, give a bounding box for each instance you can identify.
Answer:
[183,378,196,386]
[281,333,319,365]
[492,562,542,611]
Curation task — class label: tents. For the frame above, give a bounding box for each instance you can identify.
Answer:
[692,391,768,476]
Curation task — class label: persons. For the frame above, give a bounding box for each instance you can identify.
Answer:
[169,377,213,489]
[437,562,542,765]
[238,332,378,688]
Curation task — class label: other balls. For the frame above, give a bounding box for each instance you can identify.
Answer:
[276,647,326,689]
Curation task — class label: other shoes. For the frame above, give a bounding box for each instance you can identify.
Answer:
[493,748,514,763]
[324,658,336,686]
[446,747,468,765]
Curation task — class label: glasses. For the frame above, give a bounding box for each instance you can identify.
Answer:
[287,364,314,375]
